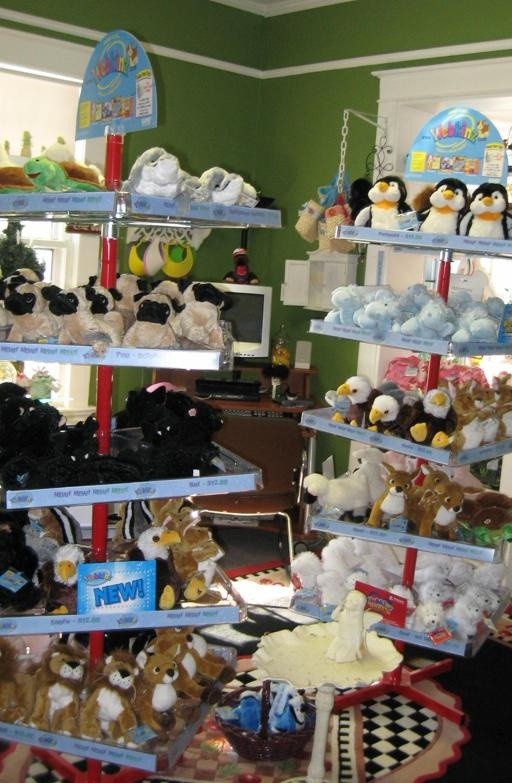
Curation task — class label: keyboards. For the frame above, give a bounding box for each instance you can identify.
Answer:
[222,409,284,418]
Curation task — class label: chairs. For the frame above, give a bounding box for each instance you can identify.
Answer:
[181,415,301,592]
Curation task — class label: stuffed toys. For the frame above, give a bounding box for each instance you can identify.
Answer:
[293,537,512,641]
[1,131,512,750]
[350,175,512,240]
[2,239,229,350]
[234,686,307,737]
[328,357,512,450]
[1,142,259,209]
[326,282,506,343]
[223,247,259,284]
[301,448,512,547]
[1,503,226,616]
[0,626,235,749]
[0,382,225,489]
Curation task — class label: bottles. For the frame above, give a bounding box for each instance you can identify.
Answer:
[270,323,290,368]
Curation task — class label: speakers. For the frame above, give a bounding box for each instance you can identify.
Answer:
[295,340,313,369]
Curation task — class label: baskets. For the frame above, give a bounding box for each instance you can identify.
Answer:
[214,678,315,761]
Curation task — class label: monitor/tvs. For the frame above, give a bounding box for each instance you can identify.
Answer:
[192,280,273,359]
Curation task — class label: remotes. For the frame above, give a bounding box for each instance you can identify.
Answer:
[272,397,296,406]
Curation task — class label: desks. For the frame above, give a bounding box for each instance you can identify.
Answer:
[152,367,324,541]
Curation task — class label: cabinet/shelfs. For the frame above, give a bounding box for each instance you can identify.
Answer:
[280,250,358,312]
[297,224,512,729]
[1,191,282,783]
